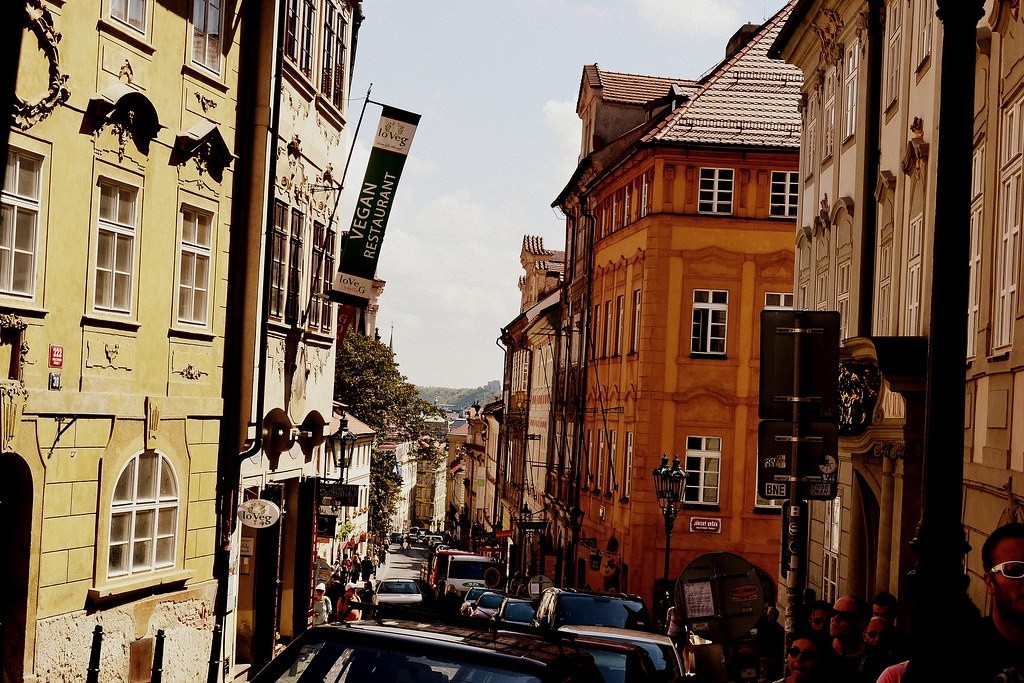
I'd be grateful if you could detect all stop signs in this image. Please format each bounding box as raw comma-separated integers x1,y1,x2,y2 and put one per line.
484,567,500,588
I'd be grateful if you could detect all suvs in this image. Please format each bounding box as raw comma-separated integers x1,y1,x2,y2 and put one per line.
248,599,607,683
389,533,403,545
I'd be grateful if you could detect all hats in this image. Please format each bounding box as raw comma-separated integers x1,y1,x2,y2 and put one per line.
871,591,897,606
315,583,326,591
345,582,358,591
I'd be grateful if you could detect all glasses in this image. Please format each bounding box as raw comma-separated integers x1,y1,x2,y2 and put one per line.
864,630,885,639
811,617,828,624
788,648,816,660
830,608,855,623
983,560,1024,578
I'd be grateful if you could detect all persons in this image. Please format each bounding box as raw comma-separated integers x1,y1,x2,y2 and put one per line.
900,523,1024,683
313,553,374,625
664,607,690,677
771,589,913,683
510,571,530,596
369,537,388,565
757,608,783,683
400,534,411,550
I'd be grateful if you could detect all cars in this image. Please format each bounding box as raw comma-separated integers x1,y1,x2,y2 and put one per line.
372,578,423,618
459,586,690,683
408,528,497,603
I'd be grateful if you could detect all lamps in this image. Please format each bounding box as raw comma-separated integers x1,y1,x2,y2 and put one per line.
317,412,358,484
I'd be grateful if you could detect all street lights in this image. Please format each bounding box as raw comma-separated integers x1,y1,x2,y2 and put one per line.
652,450,690,623
519,501,533,584
